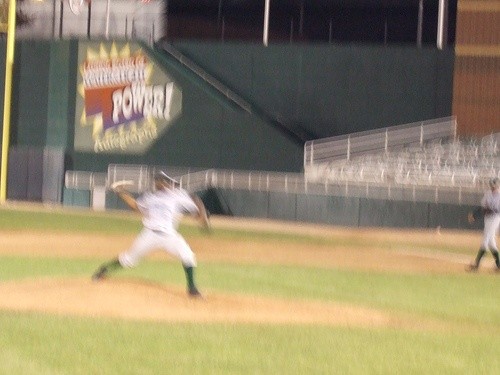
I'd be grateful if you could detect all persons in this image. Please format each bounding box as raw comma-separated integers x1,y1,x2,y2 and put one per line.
91,164,211,296
466,175,499,272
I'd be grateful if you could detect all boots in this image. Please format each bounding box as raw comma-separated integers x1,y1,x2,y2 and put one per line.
493,252,500,271
466,249,486,270
91,256,120,280
184,266,202,297
